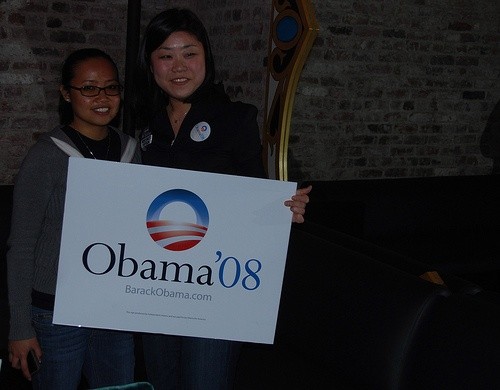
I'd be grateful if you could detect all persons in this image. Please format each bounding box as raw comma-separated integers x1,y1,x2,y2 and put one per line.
136,9,313,390
8,48,140,390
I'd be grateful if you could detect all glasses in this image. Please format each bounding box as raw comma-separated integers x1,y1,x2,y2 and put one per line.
66,83,125,98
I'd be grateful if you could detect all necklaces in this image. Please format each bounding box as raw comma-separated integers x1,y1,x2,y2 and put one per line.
169,107,190,124
76,129,111,160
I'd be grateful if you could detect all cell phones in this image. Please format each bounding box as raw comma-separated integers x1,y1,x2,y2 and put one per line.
26,349,40,377
